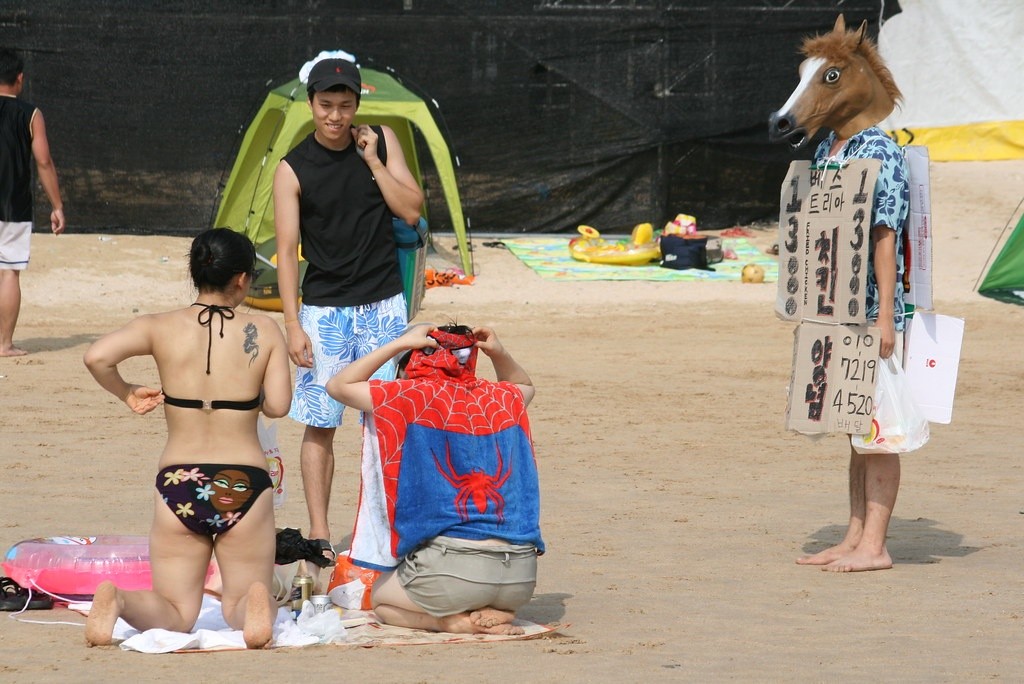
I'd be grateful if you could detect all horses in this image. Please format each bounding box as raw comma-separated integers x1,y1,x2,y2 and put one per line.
768,13,904,151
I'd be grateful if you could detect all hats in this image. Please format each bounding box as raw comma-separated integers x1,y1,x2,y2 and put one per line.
306,57,361,94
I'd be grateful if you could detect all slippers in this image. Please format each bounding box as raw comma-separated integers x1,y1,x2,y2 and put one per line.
306,538,337,567
0,576,56,613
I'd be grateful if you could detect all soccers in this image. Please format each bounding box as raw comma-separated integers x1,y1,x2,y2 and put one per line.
742,264,764,284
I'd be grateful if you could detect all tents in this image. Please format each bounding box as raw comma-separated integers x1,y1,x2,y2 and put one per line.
207,62,475,312
971,198,1024,304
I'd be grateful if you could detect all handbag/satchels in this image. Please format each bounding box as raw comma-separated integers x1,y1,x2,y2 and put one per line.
392,215,430,251
661,232,710,270
851,352,931,455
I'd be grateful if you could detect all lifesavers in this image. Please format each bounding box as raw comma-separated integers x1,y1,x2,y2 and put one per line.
569,223,662,265
3,535,215,595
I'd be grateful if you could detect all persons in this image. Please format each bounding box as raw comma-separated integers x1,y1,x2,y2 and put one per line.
768,13,930,573
273,58,424,565
0,48,67,357
83,228,292,651
324,323,545,635
